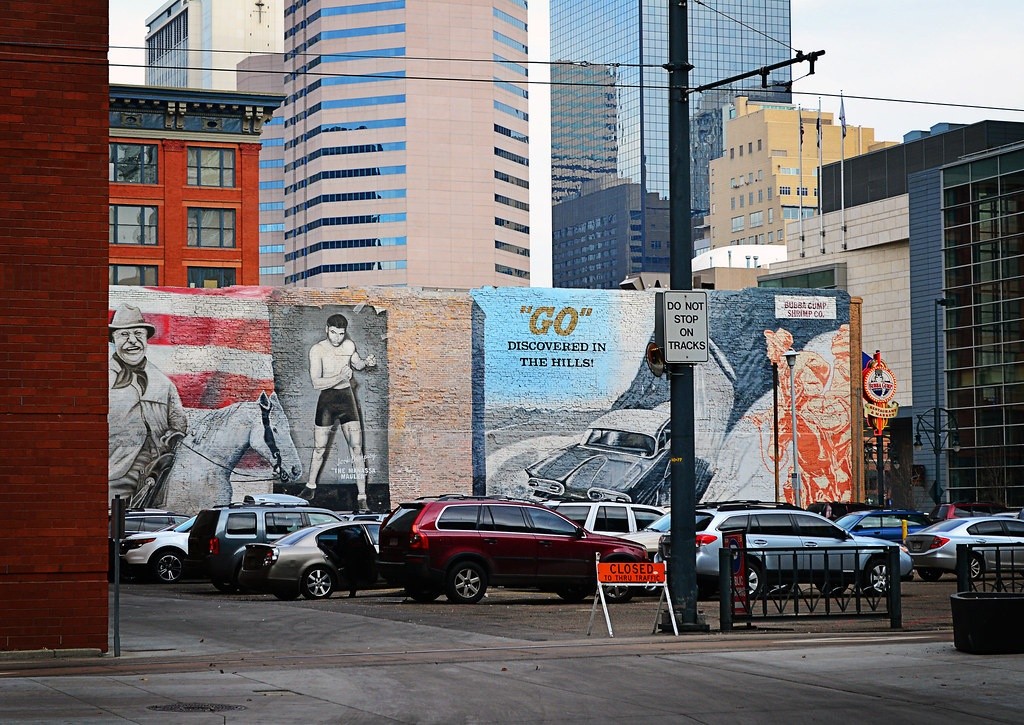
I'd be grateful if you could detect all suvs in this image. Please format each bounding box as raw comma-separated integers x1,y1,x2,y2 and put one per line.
378,493,651,604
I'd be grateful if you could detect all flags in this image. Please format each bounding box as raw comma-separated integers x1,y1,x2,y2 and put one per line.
800,118,804,145
838,90,846,139
816,118,822,148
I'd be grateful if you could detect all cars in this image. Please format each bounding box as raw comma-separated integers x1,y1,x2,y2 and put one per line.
834,509,935,541
906,517,1024,582
237,522,380,599
544,501,913,600
189,505,361,594
108,508,197,583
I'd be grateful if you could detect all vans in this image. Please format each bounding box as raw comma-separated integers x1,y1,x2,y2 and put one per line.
930,502,1024,518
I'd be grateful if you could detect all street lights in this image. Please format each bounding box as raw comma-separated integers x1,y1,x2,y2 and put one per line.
782,347,801,507
913,297,960,508
864,435,901,507
